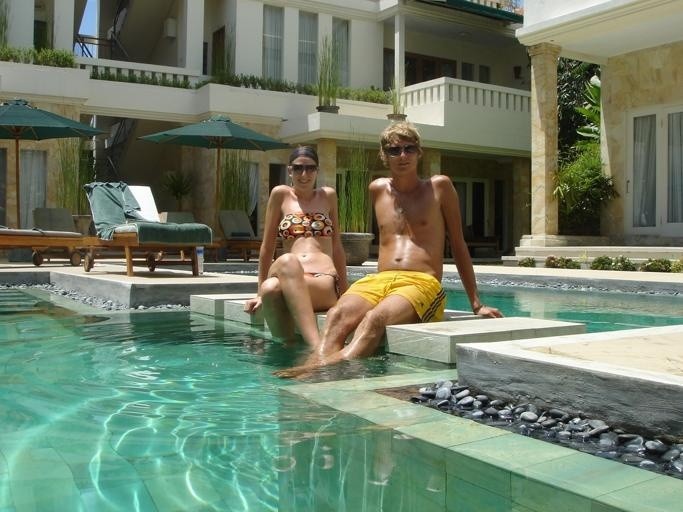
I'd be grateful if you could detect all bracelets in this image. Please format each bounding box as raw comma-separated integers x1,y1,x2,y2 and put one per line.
472,301,486,314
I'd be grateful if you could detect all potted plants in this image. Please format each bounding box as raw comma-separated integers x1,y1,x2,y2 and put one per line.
315,31,345,114
386,76,408,122
334,119,376,266
46,95,98,236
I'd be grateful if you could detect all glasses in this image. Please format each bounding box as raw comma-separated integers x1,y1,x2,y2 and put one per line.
386,145,421,156
291,163,318,175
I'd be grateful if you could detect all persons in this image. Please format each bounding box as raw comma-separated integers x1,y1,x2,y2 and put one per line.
242,145,350,351
271,365,437,492
266,120,504,379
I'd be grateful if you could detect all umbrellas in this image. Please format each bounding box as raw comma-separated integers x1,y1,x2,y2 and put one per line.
0,98,112,229
133,113,295,224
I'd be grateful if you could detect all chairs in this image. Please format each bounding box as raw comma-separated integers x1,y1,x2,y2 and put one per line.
0,182,282,277
